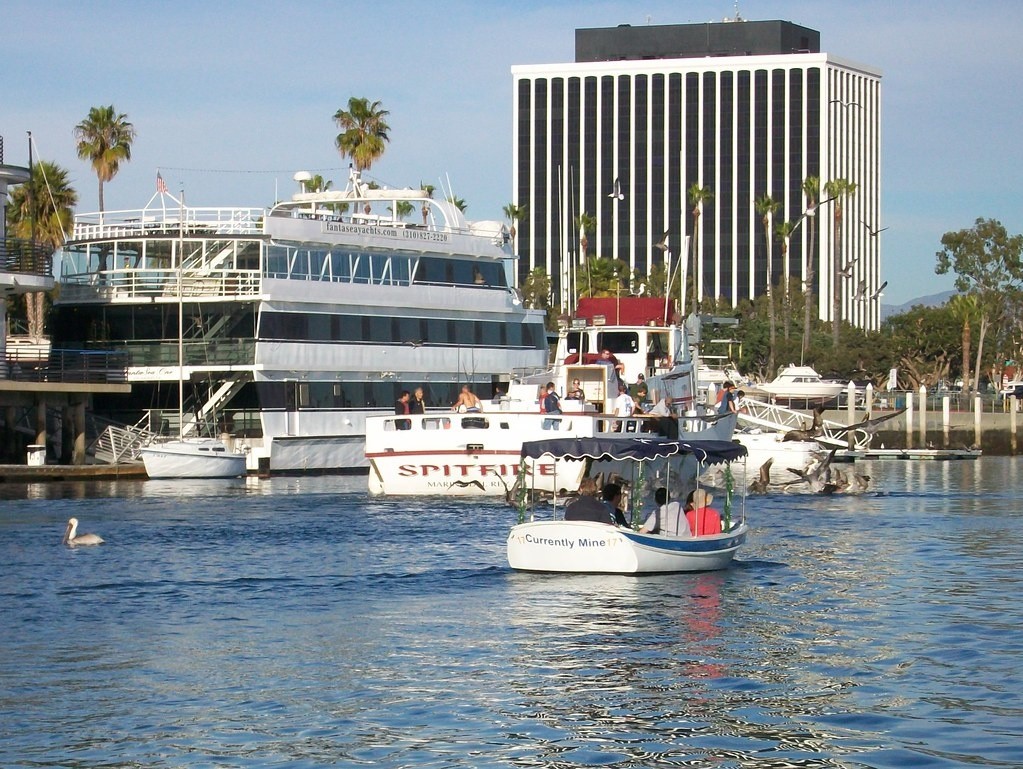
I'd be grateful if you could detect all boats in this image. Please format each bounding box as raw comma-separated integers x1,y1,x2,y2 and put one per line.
757,366,844,403
507,436,749,575
362,249,739,507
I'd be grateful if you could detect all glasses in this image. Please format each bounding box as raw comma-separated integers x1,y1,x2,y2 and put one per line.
639,378,644,380
573,382,579,385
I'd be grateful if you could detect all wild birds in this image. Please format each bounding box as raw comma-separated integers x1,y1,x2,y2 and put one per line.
962,444,982,451
780,405,827,442
829,100,863,109
858,221,887,239
773,447,869,495
472,264,486,285
627,283,647,297
827,407,909,446
491,471,540,510
786,194,838,236
403,340,428,349
880,443,885,449
446,479,485,491
924,441,935,448
62,517,107,548
607,179,624,202
837,258,889,304
746,458,773,498
652,230,671,251
939,424,966,429
188,315,209,327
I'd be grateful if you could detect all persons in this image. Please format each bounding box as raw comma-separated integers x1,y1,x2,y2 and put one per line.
713,380,745,426
595,349,627,395
564,378,585,400
648,396,677,434
611,385,635,432
538,382,563,430
394,390,410,430
492,385,507,404
565,477,613,527
408,387,427,430
450,385,484,429
683,489,722,537
601,484,630,529
636,487,693,537
628,373,649,432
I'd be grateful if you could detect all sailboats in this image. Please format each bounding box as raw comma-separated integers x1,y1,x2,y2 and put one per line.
136,188,249,479
652,236,741,389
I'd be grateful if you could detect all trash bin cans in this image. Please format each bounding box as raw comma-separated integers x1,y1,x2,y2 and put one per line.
26,445,46,466
27,484,49,500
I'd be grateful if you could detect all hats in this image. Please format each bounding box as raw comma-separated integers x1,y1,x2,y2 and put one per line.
638,373,644,379
690,489,713,510
